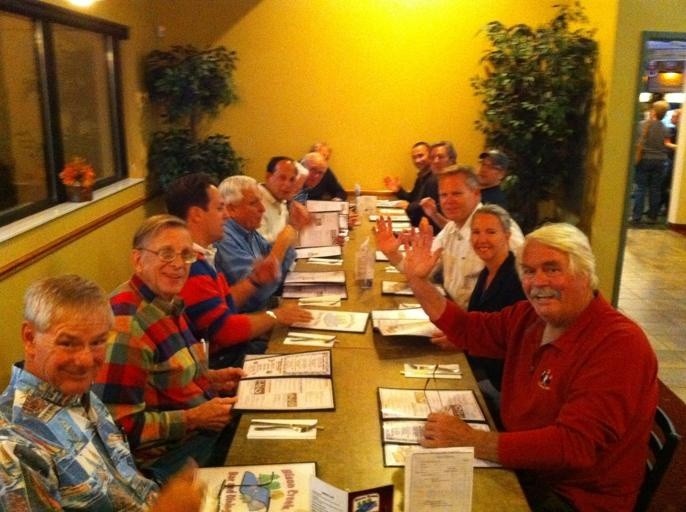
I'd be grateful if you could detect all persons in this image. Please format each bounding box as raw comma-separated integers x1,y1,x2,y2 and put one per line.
87,213,247,489
467,204,528,431
207,174,313,366
251,138,361,273
374,162,526,314
382,141,443,216
0,272,210,511
399,216,661,512
163,169,314,398
625,93,683,227
390,140,458,236
419,148,510,232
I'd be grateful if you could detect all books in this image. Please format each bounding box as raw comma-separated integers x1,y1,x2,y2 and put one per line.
372,250,413,262
367,213,412,232
191,461,318,512
230,349,336,413
375,199,402,208
379,279,448,298
278,269,350,301
374,386,507,470
369,307,443,339
286,308,369,334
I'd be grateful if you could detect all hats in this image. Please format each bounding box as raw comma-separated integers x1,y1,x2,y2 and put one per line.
479,149,510,170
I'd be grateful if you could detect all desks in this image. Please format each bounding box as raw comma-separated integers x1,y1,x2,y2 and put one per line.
214,195,531,512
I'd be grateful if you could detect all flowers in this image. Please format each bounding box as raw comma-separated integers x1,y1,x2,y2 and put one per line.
60,153,97,188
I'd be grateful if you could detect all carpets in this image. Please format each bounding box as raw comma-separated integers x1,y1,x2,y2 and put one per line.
642,377,685,512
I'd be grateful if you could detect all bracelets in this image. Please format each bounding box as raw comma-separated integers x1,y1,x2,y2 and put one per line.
264,310,281,323
246,275,263,292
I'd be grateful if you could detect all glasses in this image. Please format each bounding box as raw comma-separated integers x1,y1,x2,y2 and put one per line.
136,246,198,264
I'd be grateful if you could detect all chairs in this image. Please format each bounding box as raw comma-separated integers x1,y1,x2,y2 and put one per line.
634,406,681,512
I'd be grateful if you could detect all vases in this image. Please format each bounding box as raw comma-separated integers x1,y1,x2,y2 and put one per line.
65,185,92,202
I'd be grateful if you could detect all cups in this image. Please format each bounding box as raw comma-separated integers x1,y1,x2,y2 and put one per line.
358,255,374,289
